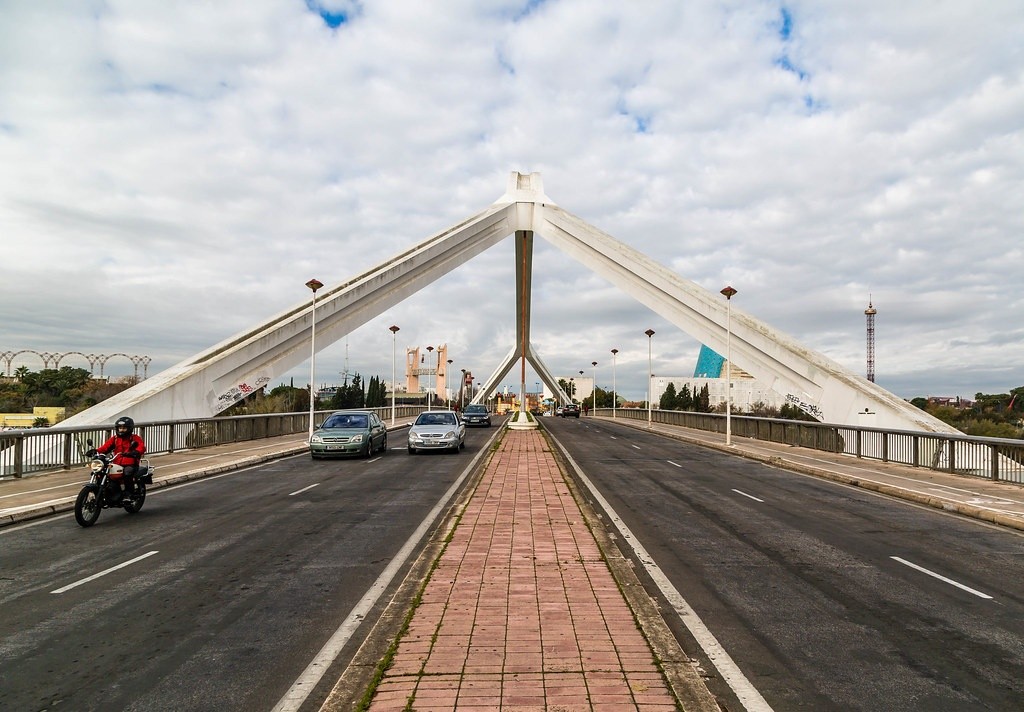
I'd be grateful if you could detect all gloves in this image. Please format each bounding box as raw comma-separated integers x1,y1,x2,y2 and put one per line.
127,450,139,458
86,449,97,457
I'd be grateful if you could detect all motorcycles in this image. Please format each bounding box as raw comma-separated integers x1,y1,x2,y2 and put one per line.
74,439,156,528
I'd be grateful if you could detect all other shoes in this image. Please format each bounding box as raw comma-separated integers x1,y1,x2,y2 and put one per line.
122,499,136,505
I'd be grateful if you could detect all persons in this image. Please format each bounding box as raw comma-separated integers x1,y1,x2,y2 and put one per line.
444,415,451,423
584,404,589,416
479,408,485,413
86,417,145,501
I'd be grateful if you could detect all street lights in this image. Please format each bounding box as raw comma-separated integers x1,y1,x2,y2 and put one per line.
610,349,619,417
579,371,584,415
592,361,597,416
645,328,655,422
304,279,323,443
569,378,574,404
388,324,400,426
426,345,434,411
720,285,738,446
559,388,562,407
447,359,481,410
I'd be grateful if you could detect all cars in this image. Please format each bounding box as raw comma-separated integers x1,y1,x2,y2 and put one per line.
555,407,563,416
310,410,388,461
407,410,466,454
459,404,492,428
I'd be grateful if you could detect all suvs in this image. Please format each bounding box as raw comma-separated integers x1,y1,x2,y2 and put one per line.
562,404,580,418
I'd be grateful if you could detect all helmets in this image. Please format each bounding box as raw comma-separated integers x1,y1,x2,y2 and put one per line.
115,417,134,438
